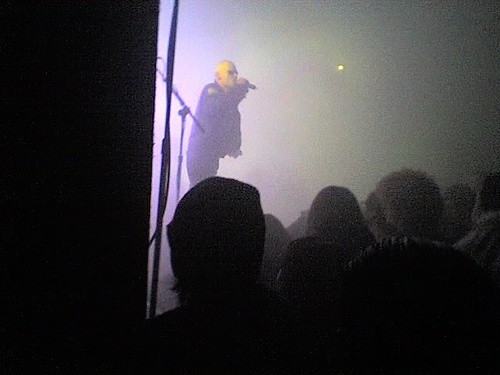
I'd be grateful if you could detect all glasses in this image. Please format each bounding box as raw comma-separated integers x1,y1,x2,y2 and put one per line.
224,70,238,74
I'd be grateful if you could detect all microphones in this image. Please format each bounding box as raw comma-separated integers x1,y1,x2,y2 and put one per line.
236,80,255,90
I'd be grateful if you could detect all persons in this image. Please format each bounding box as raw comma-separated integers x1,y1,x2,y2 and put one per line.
184,59,256,193
133,167,500,374
